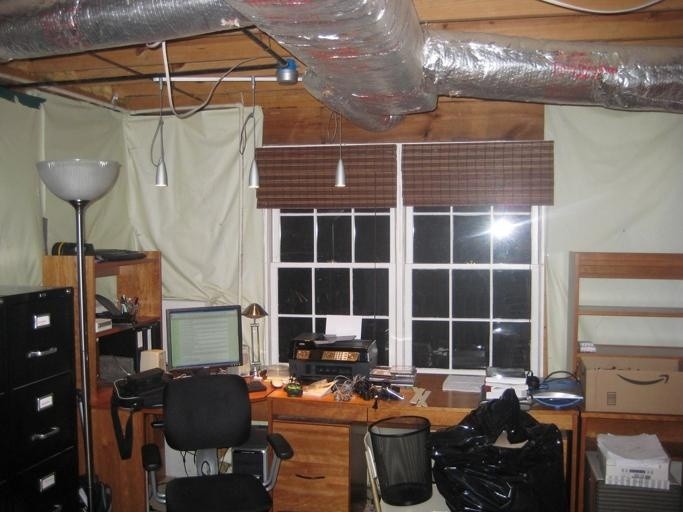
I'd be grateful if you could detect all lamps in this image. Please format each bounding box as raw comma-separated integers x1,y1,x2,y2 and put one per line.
154,81,168,191
34,158,123,512
243,303,269,381
247,76,260,190
332,111,348,188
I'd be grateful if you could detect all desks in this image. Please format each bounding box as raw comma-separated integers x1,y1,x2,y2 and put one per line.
83,381,578,512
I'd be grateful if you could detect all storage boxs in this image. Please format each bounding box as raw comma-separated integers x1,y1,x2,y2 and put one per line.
575,351,683,416
586,434,683,511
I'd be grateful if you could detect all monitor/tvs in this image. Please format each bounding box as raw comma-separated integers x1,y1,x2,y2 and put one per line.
166,305,243,377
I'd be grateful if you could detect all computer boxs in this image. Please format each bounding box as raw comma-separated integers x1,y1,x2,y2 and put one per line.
231,425,268,486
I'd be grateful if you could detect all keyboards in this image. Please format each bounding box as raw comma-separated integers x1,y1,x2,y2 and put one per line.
247,380,267,393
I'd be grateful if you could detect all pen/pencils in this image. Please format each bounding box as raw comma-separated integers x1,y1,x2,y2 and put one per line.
121,294,138,317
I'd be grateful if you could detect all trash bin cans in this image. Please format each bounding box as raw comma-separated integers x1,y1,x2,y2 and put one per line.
368,416,431,507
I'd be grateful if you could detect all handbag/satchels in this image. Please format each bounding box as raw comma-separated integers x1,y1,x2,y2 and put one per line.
111,378,167,408
426,389,563,512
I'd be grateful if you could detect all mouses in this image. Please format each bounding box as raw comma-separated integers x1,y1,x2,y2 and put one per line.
271,376,283,388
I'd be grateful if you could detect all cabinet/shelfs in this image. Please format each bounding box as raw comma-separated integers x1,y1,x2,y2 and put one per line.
0,283,83,510
568,251,683,511
42,248,164,395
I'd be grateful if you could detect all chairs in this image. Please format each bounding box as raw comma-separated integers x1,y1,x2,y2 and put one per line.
141,373,294,510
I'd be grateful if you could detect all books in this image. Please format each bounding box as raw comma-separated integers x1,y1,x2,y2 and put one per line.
443,376,484,392
483,368,529,400
